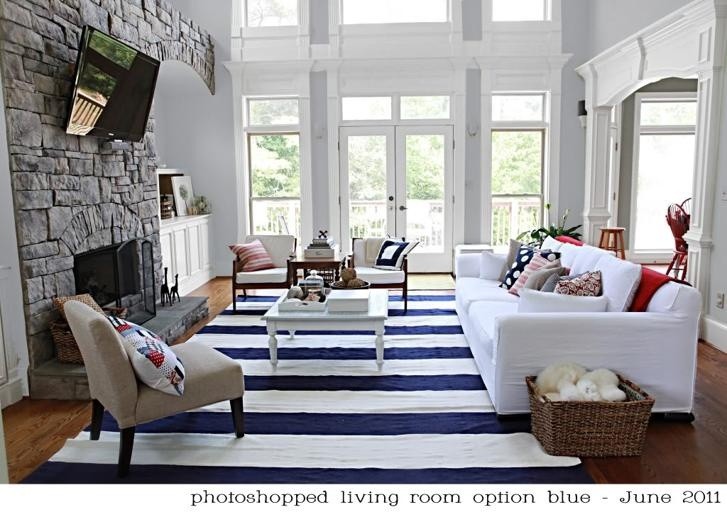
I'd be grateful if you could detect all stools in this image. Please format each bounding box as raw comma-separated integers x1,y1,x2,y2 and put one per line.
597,227,627,257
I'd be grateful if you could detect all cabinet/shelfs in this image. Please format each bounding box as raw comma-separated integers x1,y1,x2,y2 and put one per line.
157,169,217,297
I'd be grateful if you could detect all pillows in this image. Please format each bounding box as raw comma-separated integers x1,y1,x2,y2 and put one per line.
107,317,185,397
496,235,643,311
227,239,276,271
372,238,419,270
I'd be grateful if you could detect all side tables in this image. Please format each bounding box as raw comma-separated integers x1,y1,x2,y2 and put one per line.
291,256,342,286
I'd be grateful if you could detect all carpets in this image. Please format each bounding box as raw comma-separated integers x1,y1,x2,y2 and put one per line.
15,289,588,481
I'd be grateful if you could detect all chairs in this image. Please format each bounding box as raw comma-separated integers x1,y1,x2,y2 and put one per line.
63,300,245,479
233,235,296,312
665,197,697,280
348,237,407,314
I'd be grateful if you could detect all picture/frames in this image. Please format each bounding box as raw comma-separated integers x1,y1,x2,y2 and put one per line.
171,175,195,217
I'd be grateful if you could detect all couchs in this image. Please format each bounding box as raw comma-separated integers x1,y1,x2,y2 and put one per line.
455,235,703,422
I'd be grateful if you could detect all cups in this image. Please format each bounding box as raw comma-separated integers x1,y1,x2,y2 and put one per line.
308,289,322,302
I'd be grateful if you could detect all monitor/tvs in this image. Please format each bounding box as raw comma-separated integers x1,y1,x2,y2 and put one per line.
65,24,161,143
